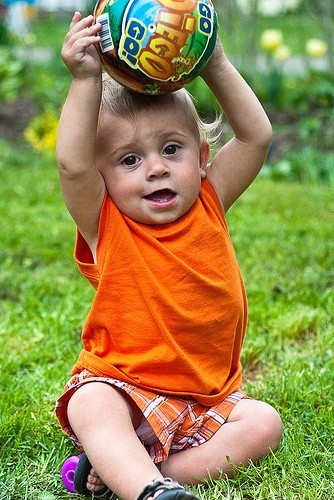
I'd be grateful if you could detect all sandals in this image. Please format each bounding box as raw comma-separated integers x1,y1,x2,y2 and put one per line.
74,453,118,499
138,478,200,500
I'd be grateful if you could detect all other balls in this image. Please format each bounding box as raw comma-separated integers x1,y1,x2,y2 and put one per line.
89,0,218,96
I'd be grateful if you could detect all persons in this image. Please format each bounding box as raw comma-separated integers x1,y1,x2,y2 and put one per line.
56,6,282,500
